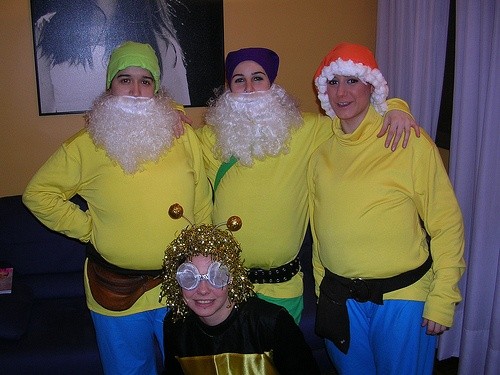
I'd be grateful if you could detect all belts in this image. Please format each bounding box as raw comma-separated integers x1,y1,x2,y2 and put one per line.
245,257,301,284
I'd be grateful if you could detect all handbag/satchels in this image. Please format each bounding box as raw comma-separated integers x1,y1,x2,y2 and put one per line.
83,243,165,311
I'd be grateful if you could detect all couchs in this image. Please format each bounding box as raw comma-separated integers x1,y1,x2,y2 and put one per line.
0,195,338,375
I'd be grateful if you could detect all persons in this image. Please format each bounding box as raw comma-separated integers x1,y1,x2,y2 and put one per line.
158,203,320,375
308,43,466,375
22,42,212,375
168,48,420,326
36,0,191,113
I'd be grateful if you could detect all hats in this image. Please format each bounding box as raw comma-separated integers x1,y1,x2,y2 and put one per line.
314,42,389,117
107,41,160,95
225,48,279,85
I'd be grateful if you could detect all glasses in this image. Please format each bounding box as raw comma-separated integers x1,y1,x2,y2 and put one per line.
175,262,230,290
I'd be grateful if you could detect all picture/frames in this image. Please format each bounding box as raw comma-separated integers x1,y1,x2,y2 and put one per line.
29,0,226,117
